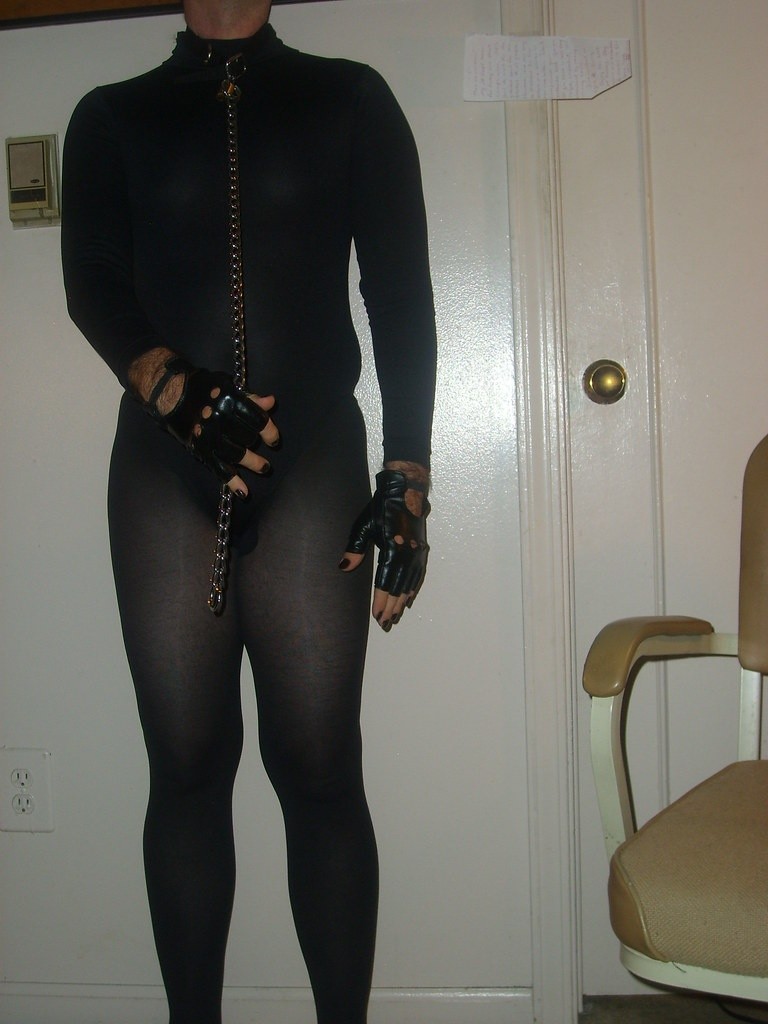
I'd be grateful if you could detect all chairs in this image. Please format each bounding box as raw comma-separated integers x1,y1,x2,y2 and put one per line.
581,436,768,1024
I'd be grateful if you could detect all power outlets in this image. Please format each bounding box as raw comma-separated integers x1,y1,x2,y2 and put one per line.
1,747,53,833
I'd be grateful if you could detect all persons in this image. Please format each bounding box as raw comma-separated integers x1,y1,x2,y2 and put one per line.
60,1,436,1024
